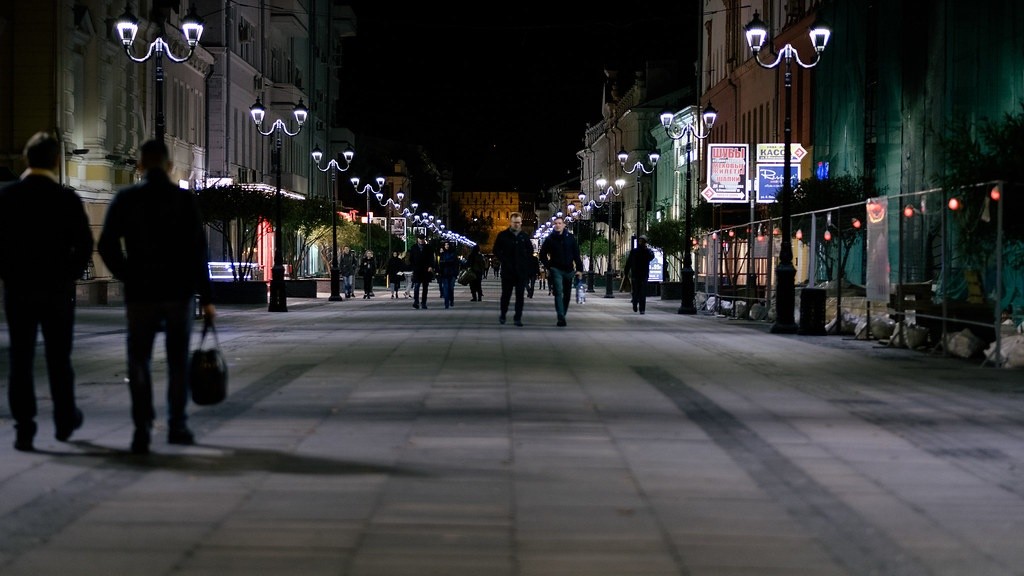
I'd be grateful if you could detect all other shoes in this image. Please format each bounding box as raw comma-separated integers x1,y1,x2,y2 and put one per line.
422,303,427,309
514,319,523,326
15,422,37,450
557,318,566,326
131,430,152,454
413,304,419,309
471,297,477,301
633,303,638,312
450,299,454,306
168,426,194,445
343,288,413,300
56,409,84,441
445,303,450,309
500,316,506,324
640,309,645,314
478,298,481,301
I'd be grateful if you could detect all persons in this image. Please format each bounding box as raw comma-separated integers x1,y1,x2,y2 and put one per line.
492,212,533,326
624,236,655,314
459,246,500,301
360,251,376,299
435,242,459,309
410,234,434,308
0,133,96,449
539,218,583,326
339,246,357,298
96,138,216,452
526,256,555,298
386,252,413,299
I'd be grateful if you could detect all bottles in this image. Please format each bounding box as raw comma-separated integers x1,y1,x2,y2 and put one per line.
576,280,586,305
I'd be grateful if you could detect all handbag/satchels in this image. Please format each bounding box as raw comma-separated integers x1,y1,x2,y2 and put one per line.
457,266,476,286
188,312,228,407
576,279,586,304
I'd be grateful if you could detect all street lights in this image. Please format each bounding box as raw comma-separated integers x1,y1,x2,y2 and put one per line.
742,6,833,335
309,141,355,302
114,0,208,144
349,170,387,296
375,187,406,289
577,186,607,292
594,173,626,299
533,200,592,289
393,199,476,273
659,100,719,316
617,142,662,303
248,93,309,313
